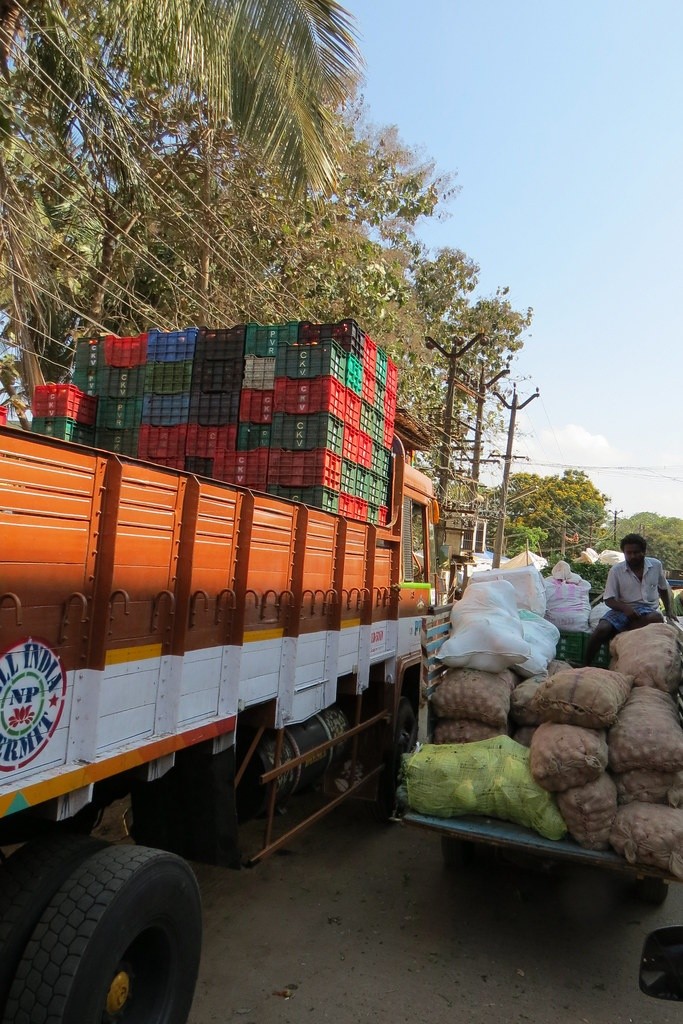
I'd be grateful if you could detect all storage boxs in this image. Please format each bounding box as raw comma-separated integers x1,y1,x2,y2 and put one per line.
146,326,198,361
95,396,143,430
100,366,145,398
141,393,190,426
185,318,397,526
144,359,192,393
141,456,185,470
556,630,582,662
582,632,610,669
74,336,104,368
71,366,100,396
138,422,185,460
32,383,97,426
103,333,148,367
31,417,94,447
94,427,138,459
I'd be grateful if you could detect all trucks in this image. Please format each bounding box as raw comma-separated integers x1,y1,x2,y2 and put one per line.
0,401,472,1024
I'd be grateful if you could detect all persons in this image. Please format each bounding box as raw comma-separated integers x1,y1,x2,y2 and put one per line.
584,532,680,670
